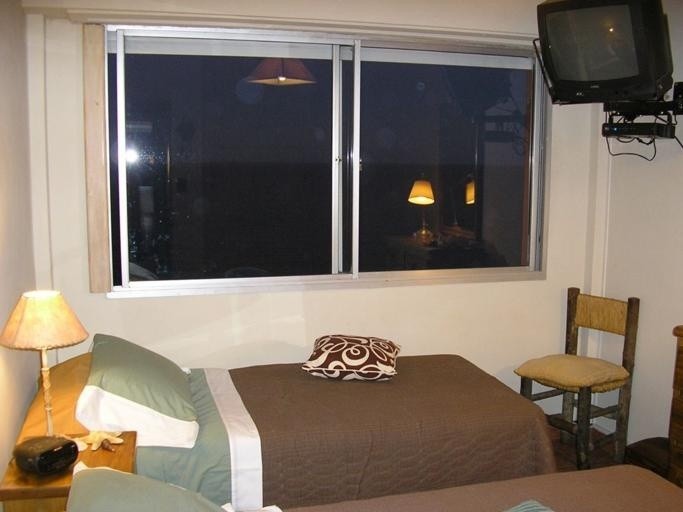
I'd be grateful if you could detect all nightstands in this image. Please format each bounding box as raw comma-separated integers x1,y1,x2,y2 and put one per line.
2,431,136,511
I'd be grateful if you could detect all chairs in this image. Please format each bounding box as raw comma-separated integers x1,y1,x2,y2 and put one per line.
514,288,640,470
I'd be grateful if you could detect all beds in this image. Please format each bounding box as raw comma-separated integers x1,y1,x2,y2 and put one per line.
134,355,556,510
286,462,681,511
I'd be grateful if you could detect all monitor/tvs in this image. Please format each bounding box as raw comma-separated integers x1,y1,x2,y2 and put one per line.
538,0,674,105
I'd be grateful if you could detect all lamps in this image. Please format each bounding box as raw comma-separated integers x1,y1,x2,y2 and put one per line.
0,288,88,442
465,181,477,223
244,60,314,88
406,179,437,245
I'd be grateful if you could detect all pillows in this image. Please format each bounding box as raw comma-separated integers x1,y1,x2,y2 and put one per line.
19,333,198,442
303,334,400,383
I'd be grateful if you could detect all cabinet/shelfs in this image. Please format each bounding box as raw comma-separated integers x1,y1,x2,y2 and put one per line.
667,323,682,487
